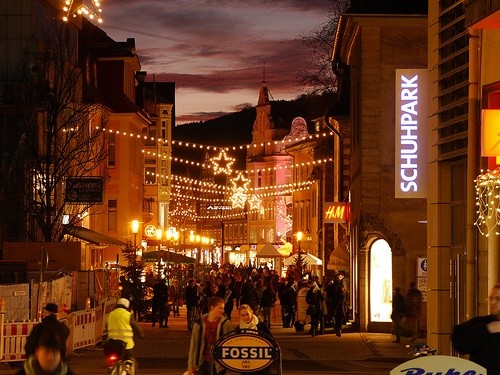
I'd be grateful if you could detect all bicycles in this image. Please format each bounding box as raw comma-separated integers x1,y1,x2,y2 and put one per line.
95,335,145,375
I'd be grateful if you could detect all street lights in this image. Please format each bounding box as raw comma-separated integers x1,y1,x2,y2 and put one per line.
154,227,165,281
127,219,145,321
293,231,304,287
166,229,215,302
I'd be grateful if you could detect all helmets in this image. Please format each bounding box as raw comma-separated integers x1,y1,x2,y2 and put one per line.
117,298,130,308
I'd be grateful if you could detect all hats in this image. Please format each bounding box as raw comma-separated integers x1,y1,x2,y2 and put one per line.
42,303,58,313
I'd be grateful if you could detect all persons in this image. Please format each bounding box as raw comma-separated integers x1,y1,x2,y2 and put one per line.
402,281,423,342
391,287,406,343
451,284,500,375
119,263,350,336
188,296,236,375
24,304,70,360
106,298,144,375
16,334,75,375
235,304,275,375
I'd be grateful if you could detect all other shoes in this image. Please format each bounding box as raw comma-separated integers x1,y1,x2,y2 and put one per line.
391,338,400,343
409,337,417,342
177,313,181,316
158,323,168,328
125,361,132,370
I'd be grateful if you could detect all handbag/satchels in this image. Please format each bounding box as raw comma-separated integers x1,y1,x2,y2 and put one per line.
306,305,316,315
